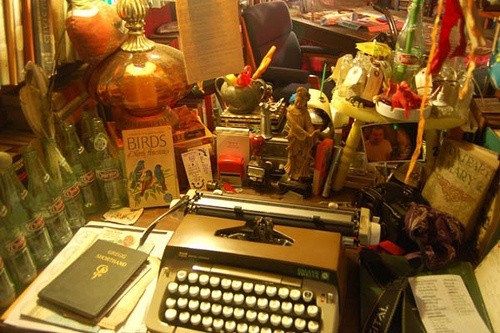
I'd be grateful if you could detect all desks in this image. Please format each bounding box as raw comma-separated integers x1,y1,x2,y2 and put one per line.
286,2,472,66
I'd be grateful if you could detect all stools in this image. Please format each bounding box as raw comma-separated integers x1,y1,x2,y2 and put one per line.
328,88,468,192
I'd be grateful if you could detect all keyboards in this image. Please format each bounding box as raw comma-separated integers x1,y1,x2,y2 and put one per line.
145,256,339,333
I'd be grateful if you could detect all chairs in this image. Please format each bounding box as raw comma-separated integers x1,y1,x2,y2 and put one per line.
243,1,348,105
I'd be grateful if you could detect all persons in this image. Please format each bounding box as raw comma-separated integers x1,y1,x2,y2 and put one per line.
392,127,416,160
283,86,321,183
364,126,392,162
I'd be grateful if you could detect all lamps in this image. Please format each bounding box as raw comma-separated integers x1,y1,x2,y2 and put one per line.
88,1,192,142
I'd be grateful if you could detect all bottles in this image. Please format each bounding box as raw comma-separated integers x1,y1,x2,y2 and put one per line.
391,0,427,95
62,122,104,214
90,117,128,209
0,199,38,285
22,146,73,248
81,111,96,153
41,134,87,229
0,152,54,265
0,254,17,309
